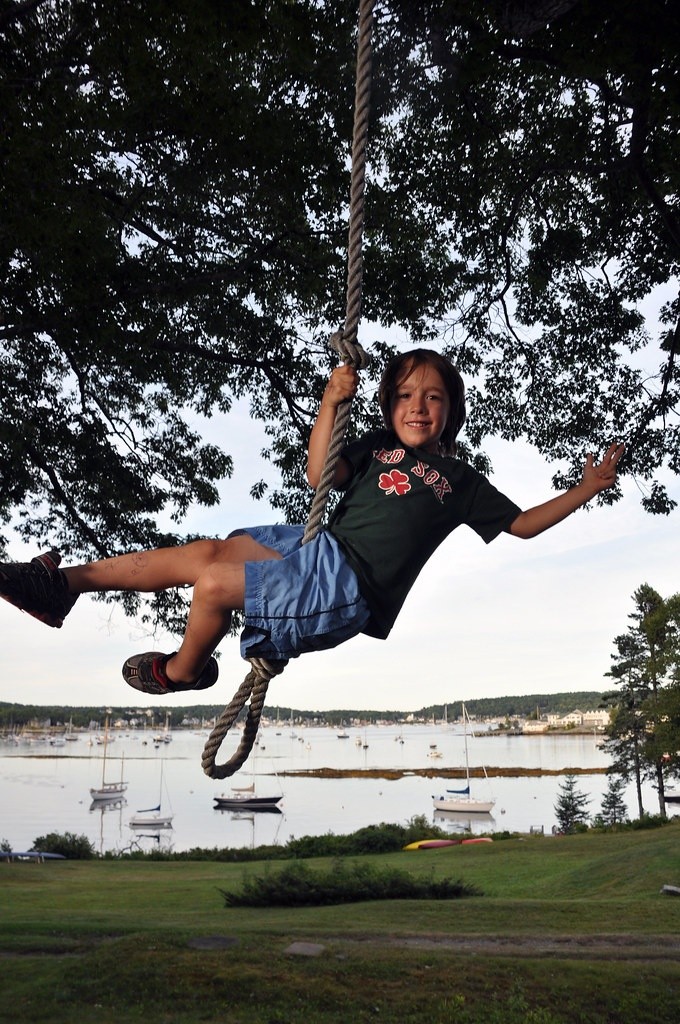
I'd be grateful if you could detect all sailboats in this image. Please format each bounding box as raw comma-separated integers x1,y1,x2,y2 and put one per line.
126,752,176,826
0,704,503,754
128,822,175,854
213,718,285,807
429,701,499,814
89,796,129,855
212,803,285,849
89,713,130,802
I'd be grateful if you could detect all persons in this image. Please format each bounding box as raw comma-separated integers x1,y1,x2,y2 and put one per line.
0,348,625,693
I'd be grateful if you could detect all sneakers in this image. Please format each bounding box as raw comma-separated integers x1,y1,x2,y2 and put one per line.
122,651,219,695
0,551,81,628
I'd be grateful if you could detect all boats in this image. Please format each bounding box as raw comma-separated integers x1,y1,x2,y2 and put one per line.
595,733,680,804
